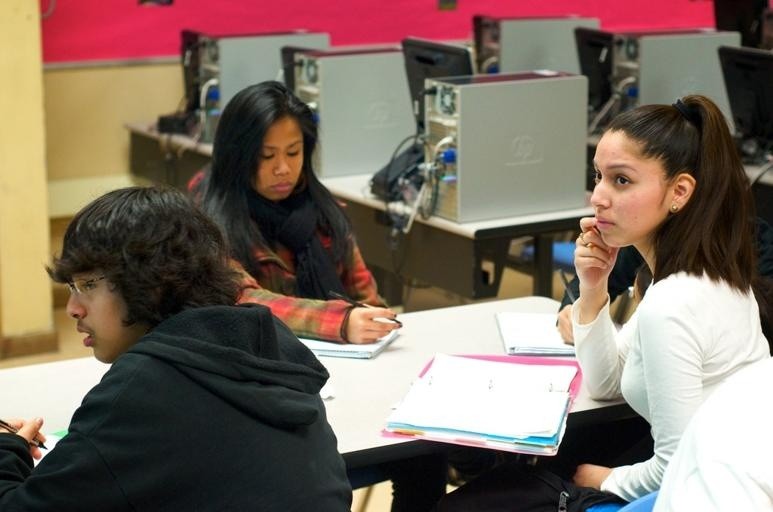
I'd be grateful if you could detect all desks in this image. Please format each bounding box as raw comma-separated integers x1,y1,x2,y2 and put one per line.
0,120,772,511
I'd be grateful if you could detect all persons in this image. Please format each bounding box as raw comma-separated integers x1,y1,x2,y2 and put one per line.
434,92,771,511
556,244,642,345
0,184,352,511
186,81,448,511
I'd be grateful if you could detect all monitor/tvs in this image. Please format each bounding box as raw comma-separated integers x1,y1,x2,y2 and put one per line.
281,46,314,91
371,37,474,201
159,29,202,135
717,45,773,165
574,28,615,125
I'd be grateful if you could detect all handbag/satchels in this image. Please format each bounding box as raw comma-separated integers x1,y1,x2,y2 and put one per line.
371,143,424,201
435,462,616,512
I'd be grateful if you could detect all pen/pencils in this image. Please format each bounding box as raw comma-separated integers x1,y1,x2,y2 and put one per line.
328,290,402,324
0,419,48,449
559,268,576,304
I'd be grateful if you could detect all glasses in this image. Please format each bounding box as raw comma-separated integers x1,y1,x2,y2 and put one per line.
67,276,108,294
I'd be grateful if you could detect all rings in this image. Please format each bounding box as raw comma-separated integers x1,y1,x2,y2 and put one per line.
578,232,587,246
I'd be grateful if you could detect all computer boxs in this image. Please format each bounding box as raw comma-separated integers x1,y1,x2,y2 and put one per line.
197,30,330,143
608,27,742,137
472,15,600,75
292,43,418,180
421,71,591,223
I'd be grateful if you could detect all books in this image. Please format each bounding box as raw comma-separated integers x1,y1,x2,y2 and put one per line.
383,350,581,458
496,311,586,357
296,319,400,359
